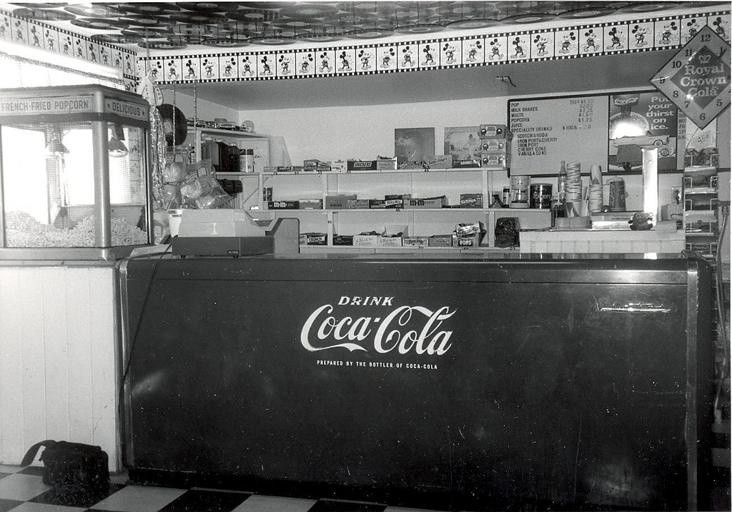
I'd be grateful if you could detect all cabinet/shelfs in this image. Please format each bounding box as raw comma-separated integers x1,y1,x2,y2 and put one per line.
184,122,715,259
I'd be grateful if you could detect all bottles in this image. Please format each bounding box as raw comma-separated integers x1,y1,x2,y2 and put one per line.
224,143,253,173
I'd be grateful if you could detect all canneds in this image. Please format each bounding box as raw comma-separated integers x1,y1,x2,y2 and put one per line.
240,155,255,174
503,175,553,209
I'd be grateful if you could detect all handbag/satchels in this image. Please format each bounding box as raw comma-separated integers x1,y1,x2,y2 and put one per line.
20,439,109,487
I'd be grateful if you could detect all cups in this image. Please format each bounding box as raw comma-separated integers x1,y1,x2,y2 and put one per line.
564,161,626,217
